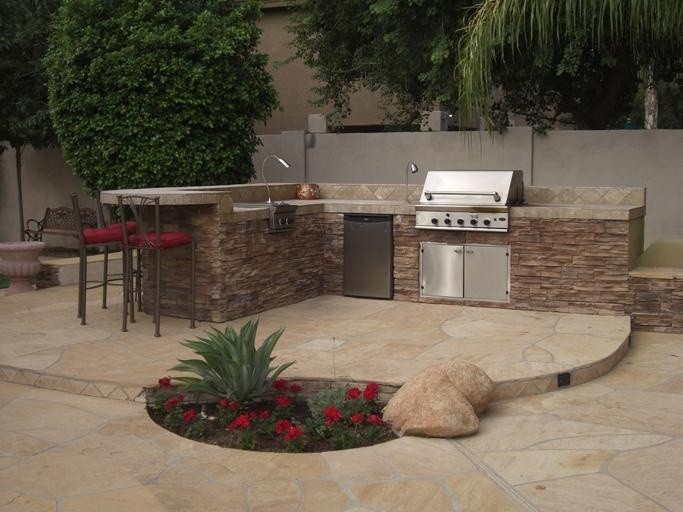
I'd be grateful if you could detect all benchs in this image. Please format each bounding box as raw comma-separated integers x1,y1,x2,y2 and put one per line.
24,206,97,242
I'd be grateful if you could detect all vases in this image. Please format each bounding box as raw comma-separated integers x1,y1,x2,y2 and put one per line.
0,241,46,296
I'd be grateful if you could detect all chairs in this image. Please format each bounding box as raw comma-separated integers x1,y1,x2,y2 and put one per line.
69,189,195,337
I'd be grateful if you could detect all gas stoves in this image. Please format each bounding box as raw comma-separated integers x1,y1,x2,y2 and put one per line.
414,170,526,234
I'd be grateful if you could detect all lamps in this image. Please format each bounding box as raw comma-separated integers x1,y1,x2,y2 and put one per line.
404,160,418,203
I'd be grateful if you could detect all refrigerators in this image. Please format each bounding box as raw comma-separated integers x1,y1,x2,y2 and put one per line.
343,214,393,299
